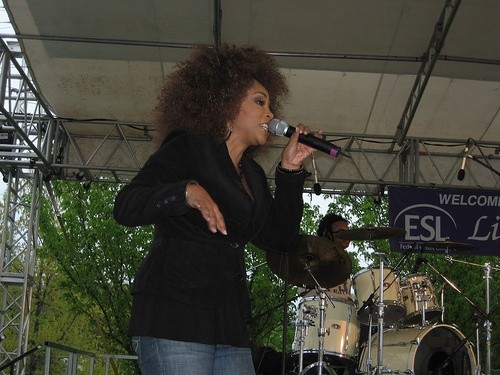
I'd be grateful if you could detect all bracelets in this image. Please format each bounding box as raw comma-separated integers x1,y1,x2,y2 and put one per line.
278,159,305,174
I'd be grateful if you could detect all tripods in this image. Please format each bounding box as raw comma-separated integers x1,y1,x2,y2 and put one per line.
299,264,337,375
370,235,397,375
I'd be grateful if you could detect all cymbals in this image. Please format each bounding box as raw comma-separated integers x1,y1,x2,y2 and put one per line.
266,234,353,289
335,226,407,240
427,240,473,248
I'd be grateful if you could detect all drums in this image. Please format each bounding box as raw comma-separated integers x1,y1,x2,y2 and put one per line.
352,265,405,326
358,323,476,375
291,296,361,369
399,273,442,328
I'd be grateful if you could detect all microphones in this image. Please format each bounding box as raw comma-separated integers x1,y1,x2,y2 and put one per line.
458,140,470,181
268,119,340,157
311,152,321,196
412,245,426,274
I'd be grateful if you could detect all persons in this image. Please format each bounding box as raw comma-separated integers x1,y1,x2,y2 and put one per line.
113,43,324,375
298,215,350,300
